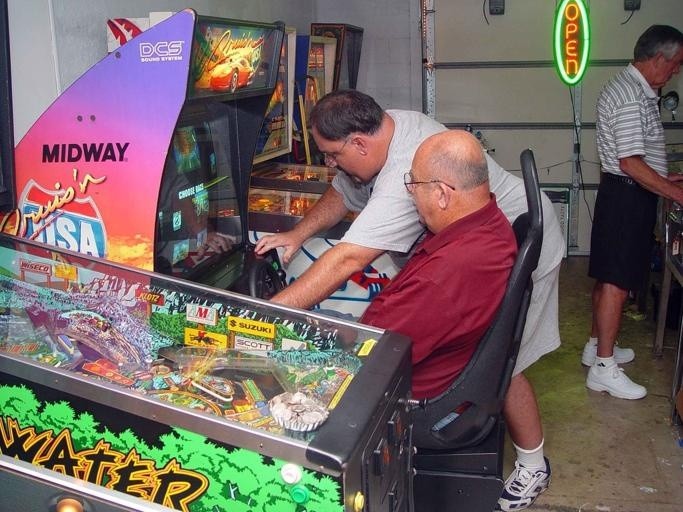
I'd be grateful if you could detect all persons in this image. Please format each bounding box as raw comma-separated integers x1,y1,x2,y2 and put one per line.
582,25,683,400
311,130,519,431
162,159,233,260
254,89,566,512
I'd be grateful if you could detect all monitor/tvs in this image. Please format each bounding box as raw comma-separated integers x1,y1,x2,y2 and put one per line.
156,102,244,281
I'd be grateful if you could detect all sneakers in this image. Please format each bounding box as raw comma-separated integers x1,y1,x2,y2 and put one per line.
586,364,648,402
496,455,551,511
581,339,637,368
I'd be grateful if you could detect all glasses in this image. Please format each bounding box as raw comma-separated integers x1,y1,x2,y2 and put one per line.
403,172,457,195
320,139,350,168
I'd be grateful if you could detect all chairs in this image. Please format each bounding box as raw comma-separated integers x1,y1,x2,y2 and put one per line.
371,146,545,509
